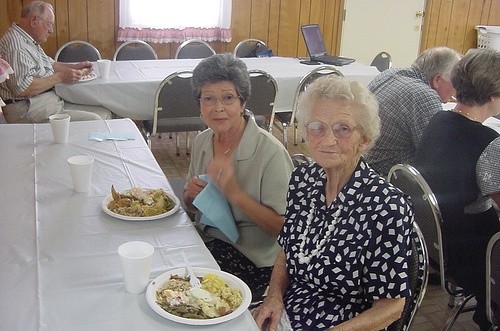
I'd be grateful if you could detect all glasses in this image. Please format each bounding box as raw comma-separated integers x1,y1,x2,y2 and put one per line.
197,93,240,106
305,121,361,138
37,16,54,29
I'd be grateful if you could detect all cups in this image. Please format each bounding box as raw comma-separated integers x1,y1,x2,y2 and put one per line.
68,155,94,192
49,114,71,143
117,241,155,295
96,60,111,79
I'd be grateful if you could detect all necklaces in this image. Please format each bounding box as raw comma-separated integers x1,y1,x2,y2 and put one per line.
453,108,481,123
222,146,233,155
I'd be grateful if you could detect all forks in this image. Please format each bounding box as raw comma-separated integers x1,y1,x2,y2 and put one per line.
181,251,202,288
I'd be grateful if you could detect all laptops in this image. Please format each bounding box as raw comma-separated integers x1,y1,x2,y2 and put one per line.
300,24,354,66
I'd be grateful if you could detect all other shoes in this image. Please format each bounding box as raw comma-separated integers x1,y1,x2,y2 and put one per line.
426,271,442,286
472,301,500,331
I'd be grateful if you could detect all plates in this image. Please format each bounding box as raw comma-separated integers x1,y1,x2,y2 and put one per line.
145,267,253,326
79,74,97,81
101,187,181,221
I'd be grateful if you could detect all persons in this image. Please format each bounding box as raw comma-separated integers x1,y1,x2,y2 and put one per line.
357,47,463,175
183,52,294,301
414,47,500,331
0,1,112,125
251,73,414,331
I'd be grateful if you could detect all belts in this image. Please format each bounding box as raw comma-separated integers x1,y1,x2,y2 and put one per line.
4,86,55,105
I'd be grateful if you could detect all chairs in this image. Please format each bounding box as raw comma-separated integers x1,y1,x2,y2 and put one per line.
53,38,500,331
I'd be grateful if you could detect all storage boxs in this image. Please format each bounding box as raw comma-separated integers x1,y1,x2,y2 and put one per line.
473,24,500,51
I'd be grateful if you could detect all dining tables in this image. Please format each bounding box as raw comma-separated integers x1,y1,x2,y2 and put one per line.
54,55,381,155
0,118,259,331
